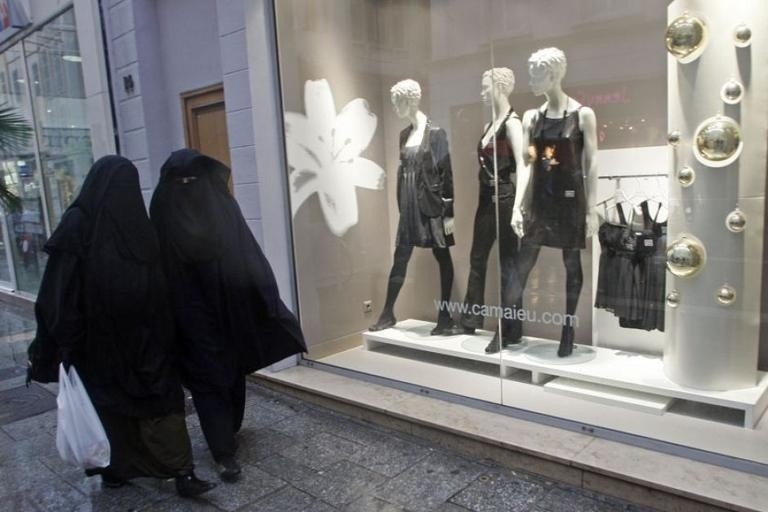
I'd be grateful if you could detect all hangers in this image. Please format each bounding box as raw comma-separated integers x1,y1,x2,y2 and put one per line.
587,172,669,227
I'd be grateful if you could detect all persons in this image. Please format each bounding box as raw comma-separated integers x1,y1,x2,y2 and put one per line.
368,79,456,336
508,45,598,358
443,66,523,352
150,148,306,483
25,154,215,496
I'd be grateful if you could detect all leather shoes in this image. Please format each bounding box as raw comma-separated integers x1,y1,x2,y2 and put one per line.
174,476,217,497
99,471,123,487
218,457,244,477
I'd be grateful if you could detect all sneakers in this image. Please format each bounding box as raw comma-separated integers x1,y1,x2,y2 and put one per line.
368,318,398,332
443,326,475,335
430,318,453,335
486,333,509,353
557,326,575,358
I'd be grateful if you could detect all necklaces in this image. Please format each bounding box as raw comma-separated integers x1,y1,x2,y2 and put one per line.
540,94,568,160
